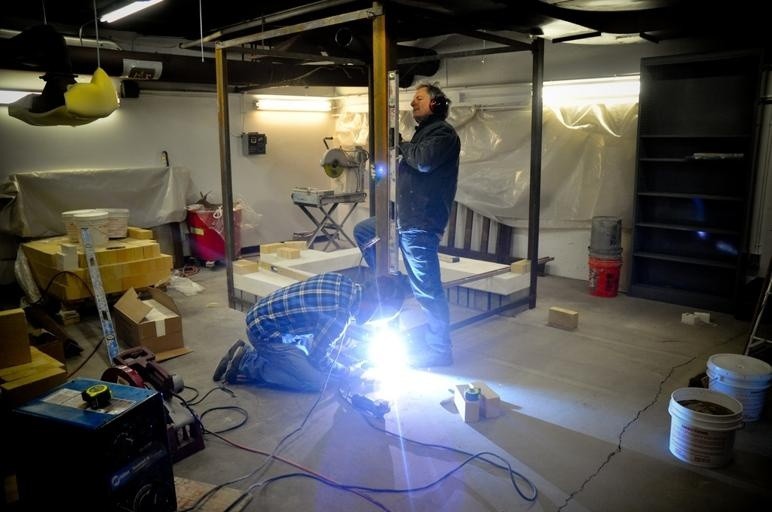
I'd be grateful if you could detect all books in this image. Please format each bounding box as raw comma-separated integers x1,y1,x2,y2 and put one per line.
113,286,196,362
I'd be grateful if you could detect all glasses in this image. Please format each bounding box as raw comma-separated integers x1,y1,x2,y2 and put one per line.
98,0,163,23
253,100,334,111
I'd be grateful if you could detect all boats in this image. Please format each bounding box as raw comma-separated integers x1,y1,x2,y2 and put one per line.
119,79,140,99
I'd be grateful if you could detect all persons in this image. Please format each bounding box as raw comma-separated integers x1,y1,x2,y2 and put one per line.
210,273,404,395
354,79,462,371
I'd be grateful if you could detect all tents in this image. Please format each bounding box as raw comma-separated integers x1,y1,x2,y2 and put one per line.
430,95,450,117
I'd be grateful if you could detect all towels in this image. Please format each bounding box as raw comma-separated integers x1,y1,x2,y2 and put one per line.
187,202,242,262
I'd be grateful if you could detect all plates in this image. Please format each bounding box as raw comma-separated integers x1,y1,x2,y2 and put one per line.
627,46,765,313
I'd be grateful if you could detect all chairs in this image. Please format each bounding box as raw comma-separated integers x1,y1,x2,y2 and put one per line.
212,336,246,382
408,353,453,368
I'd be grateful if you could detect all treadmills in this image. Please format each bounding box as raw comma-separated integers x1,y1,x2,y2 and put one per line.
81,384,112,408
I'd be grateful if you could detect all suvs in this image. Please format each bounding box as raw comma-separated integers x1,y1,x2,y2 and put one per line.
667,387,745,469
589,256,624,297
706,353,772,421
61,209,94,243
97,207,130,238
72,212,109,244
588,215,624,260
